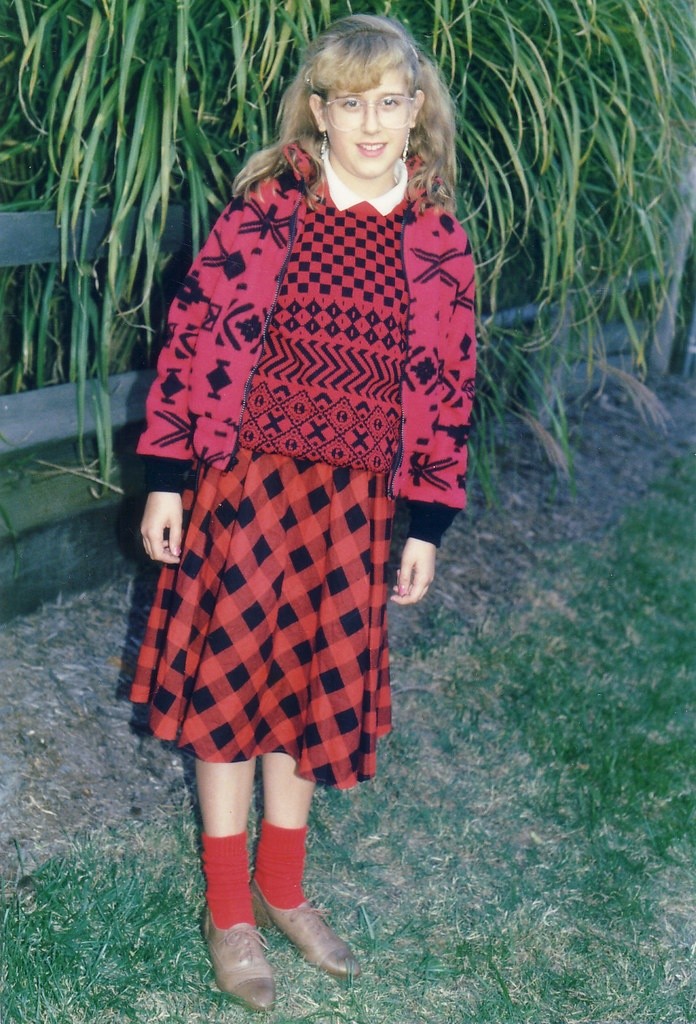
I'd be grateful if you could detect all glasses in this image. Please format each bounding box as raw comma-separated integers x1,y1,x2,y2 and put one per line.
319,95,415,132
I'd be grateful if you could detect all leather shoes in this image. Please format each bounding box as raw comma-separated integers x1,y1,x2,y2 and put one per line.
248,879,361,980
201,908,275,1010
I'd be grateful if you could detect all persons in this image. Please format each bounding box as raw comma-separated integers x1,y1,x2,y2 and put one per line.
126,14,477,1013
